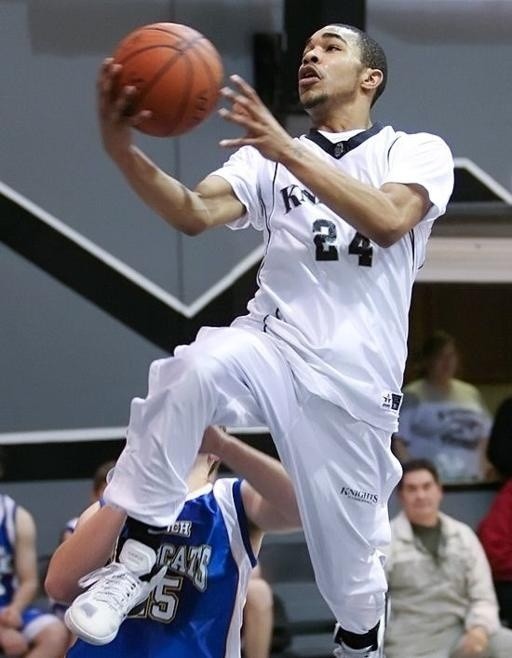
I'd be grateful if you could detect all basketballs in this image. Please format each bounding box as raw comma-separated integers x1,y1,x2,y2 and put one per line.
110,21,224,138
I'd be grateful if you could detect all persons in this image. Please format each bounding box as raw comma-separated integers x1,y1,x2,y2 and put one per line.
379,460,511,658
41,424,304,657
62,22,456,657
391,328,495,484
238,557,274,657
471,391,512,630
64,456,120,537
1,492,73,658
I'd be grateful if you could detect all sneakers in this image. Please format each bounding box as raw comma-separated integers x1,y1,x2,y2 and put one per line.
63,537,171,646
333,596,390,658
